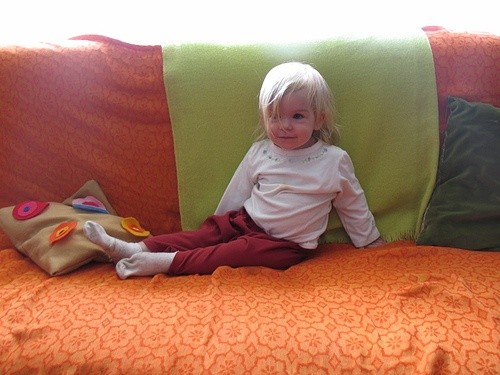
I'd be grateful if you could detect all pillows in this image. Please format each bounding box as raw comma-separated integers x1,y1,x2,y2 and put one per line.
0,178,156,276
416,96,500,252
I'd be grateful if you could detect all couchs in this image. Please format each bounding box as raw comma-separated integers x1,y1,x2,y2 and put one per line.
0,24,500,375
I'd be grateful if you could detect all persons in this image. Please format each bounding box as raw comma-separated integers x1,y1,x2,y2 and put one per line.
86,61,385,279
